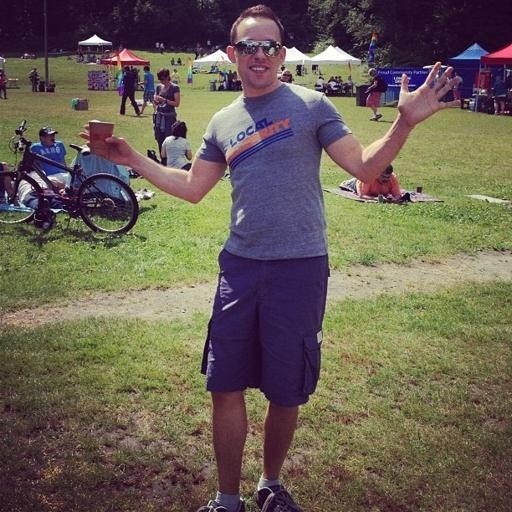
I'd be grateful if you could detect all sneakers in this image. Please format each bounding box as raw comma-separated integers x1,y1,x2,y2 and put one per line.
196,497,248,512
373,113,382,119
254,483,302,512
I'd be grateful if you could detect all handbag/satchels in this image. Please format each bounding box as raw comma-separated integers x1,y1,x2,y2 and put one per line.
374,77,387,92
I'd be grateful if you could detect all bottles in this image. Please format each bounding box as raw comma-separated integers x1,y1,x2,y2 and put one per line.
73,174,80,198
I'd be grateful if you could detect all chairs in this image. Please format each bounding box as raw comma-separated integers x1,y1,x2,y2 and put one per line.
315,82,355,97
211,79,244,92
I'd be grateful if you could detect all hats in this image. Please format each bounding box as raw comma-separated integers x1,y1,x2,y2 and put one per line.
38,126,58,137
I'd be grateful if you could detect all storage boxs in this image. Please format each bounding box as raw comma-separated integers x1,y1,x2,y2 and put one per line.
73,99,88,110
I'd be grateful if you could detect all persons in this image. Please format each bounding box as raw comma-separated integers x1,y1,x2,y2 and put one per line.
339,163,402,202
278,64,512,124
28,68,41,92
118,37,243,173
75,45,113,68
0,68,9,99
0,125,71,218
79,5,463,512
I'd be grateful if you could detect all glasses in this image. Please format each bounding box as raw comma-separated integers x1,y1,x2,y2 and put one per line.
233,38,284,56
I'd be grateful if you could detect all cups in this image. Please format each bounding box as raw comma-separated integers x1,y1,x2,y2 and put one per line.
89,121,115,156
416,187,421,193
378,195,383,204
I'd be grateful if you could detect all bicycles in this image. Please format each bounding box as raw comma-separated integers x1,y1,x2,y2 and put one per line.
0,121,142,238
